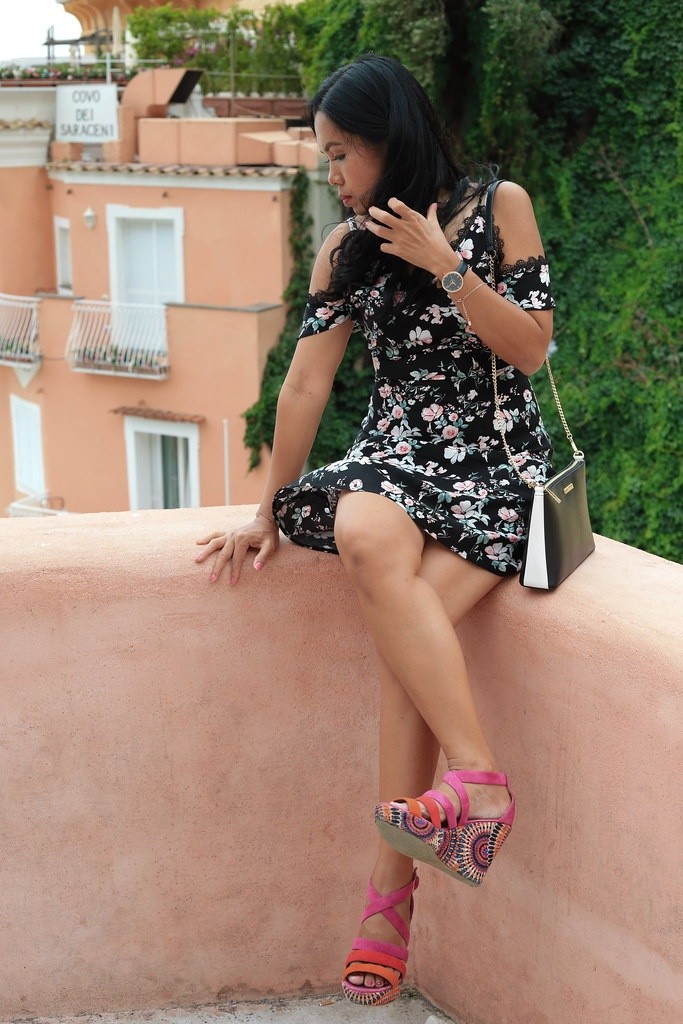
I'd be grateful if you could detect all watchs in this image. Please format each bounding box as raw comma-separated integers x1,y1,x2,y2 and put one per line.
435,260,468,295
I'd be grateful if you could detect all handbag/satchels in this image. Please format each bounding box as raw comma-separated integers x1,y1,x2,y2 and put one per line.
517,449,596,592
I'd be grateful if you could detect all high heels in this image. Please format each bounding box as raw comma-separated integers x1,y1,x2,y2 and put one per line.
374,770,513,885
343,870,416,1003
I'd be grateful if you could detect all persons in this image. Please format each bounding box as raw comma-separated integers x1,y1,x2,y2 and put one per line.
191,59,558,1008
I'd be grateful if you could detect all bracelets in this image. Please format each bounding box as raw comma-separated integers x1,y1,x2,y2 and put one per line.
451,282,488,329
